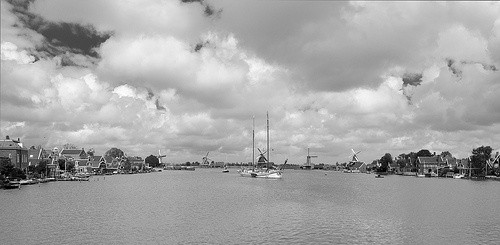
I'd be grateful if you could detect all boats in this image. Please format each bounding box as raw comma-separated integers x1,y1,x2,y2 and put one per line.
222,170,230,173
375,175,384,178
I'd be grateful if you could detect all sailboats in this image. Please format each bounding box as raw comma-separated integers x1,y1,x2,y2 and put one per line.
238,112,283,180
